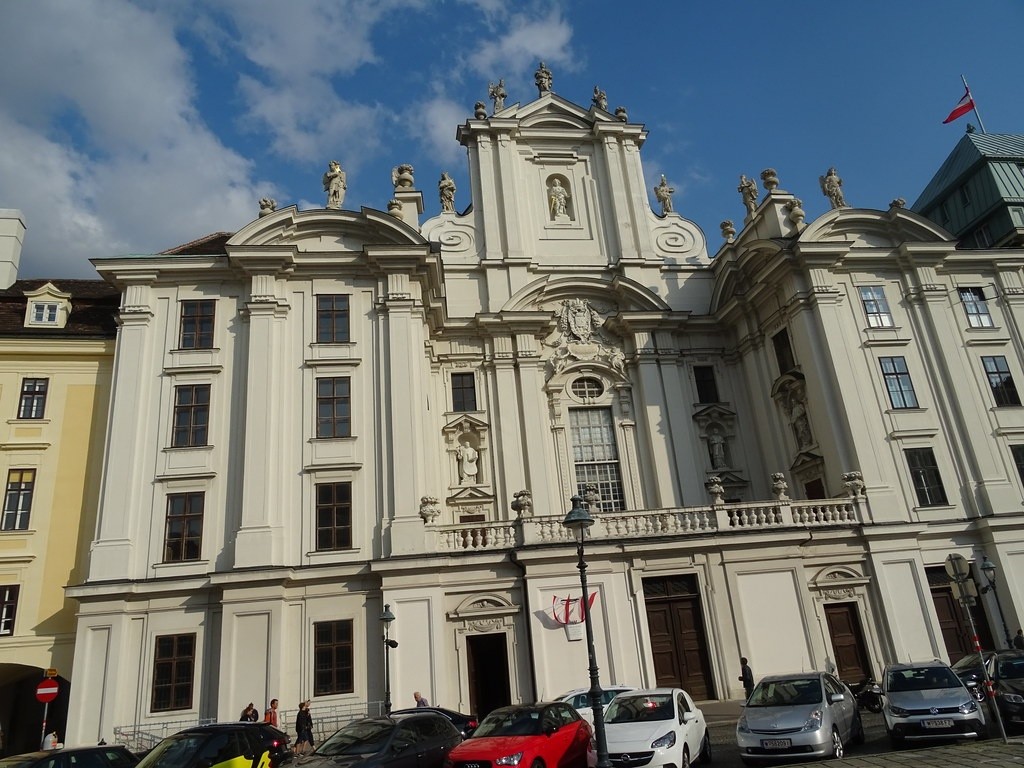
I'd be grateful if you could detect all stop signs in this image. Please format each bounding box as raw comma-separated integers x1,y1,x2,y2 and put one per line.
35,680,60,702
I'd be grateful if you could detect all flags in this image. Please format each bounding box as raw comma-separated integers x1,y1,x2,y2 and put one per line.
943,87,976,124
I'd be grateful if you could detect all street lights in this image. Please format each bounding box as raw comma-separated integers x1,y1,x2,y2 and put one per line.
981,555,1016,649
565,494,615,768
379,603,395,713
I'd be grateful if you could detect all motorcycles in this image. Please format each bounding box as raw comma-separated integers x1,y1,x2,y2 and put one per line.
830,667,882,713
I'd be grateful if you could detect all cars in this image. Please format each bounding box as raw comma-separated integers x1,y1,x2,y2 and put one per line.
588,689,712,768
396,707,479,739
278,713,460,768
448,702,593,767
506,689,657,749
735,673,865,764
0,722,294,768
984,648,1024,731
951,651,997,703
881,660,989,750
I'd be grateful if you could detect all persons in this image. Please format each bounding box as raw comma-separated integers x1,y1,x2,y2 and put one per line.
658,180,673,212
460,442,478,481
554,350,569,374
293,700,316,757
324,161,347,208
1014,629,1024,650
608,347,625,374
534,62,552,90
824,168,846,206
98,738,107,745
440,173,456,212
265,699,279,727
492,80,507,112
239,704,259,722
710,428,726,469
594,87,606,109
739,175,759,214
739,658,754,706
550,179,570,216
414,692,429,707
788,399,812,448
